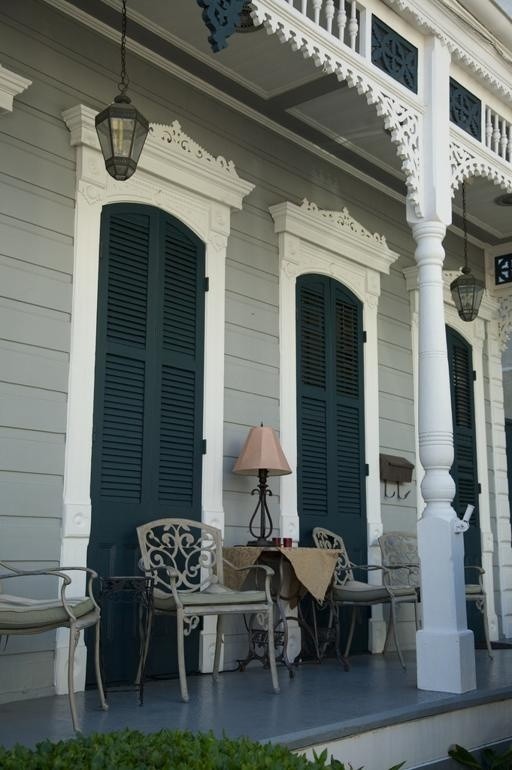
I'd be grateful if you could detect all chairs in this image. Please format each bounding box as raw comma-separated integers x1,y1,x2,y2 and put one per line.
133,518,281,703
312,526,420,671
0,561,109,734
378,534,495,661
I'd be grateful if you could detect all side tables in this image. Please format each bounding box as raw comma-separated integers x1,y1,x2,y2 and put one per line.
93,573,154,707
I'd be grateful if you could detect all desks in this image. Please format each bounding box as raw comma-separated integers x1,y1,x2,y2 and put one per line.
211,546,343,680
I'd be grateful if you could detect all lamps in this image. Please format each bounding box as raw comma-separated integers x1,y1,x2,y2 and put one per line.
450,180,485,322
94,0,150,182
232,421,293,548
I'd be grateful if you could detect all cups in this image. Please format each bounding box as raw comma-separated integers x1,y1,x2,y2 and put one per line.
272,537,293,547
